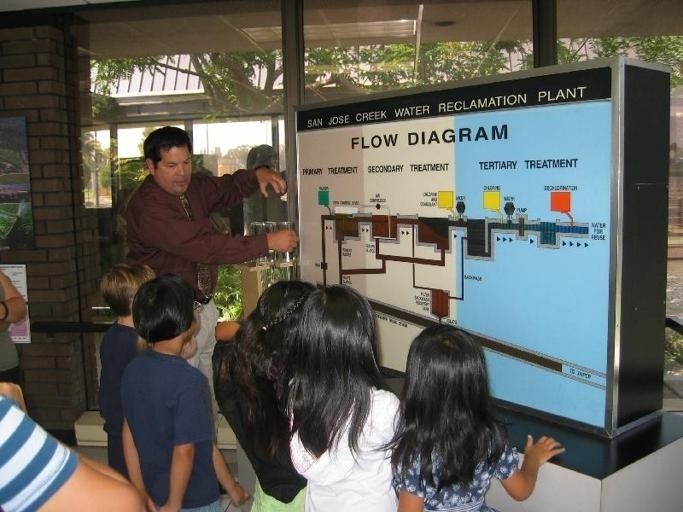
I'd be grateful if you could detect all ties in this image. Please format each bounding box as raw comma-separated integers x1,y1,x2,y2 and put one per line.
180,195,213,299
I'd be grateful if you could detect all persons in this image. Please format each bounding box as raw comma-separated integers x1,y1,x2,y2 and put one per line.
97,259,401,510
1,259,143,512
123,126,300,444
373,328,569,510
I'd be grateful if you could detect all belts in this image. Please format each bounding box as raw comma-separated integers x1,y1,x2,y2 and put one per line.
194,295,212,304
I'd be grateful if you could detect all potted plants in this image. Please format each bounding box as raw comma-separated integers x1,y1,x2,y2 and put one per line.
215,264,242,341
